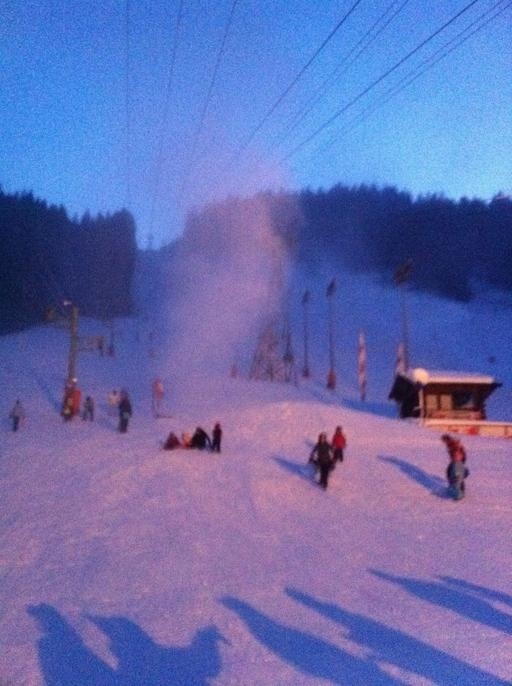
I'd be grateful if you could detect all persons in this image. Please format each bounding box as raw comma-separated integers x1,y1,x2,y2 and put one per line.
162,421,223,454
330,426,347,464
106,389,121,416
81,394,96,421
117,392,134,433
447,449,470,499
307,433,335,490
9,399,23,433
153,376,167,418
441,434,466,494
